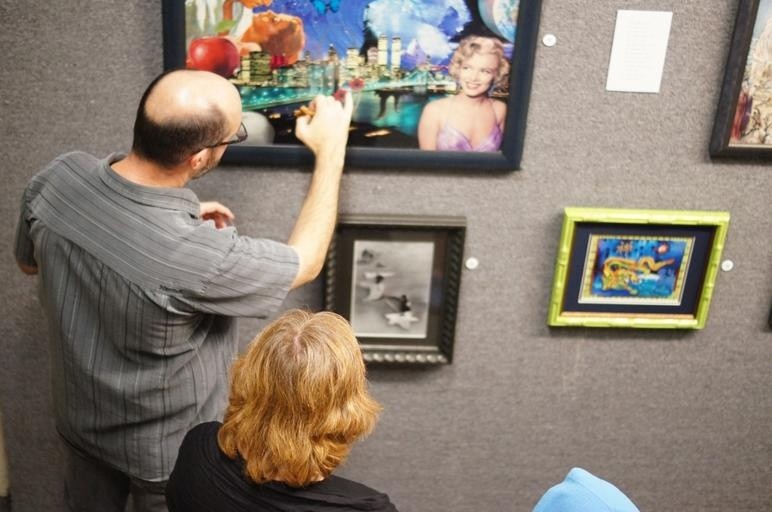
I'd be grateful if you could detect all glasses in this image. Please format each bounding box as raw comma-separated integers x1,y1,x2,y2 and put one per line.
191,122,248,155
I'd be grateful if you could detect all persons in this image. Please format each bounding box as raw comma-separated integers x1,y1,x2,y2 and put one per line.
416,35,510,152
16,68,351,508
166,311,401,511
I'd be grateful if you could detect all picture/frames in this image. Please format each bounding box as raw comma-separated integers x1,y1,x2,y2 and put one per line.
156,0,542,177
545,204,730,335
323,211,466,369
705,0,772,163
